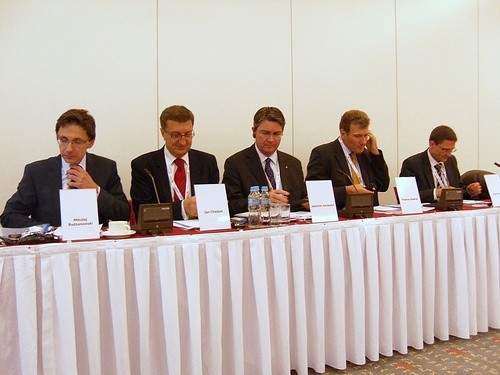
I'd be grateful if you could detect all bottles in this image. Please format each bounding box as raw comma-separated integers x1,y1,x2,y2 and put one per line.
248,186,270,228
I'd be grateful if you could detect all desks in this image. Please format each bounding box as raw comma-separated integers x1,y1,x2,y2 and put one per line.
0,200,499,375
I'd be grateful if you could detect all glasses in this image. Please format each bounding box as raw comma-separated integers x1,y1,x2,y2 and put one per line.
164,129,195,139
57,134,90,147
255,128,283,140
437,145,456,153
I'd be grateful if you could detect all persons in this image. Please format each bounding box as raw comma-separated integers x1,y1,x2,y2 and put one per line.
0,109,130,231
305,110,391,208
222,107,310,217
399,126,482,203
130,106,220,225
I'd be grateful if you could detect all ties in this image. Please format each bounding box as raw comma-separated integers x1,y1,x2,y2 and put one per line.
434,163,444,189
174,159,187,203
264,157,276,190
69,165,82,189
349,153,360,185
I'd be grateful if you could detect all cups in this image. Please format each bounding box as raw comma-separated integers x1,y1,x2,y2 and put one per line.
269,203,290,227
108,221,130,234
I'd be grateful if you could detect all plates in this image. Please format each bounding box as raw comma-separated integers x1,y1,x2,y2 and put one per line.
101,230,136,238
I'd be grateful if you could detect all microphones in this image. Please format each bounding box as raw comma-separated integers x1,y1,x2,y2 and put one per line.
336,168,359,193
436,166,449,186
495,163,500,167
144,168,160,204
